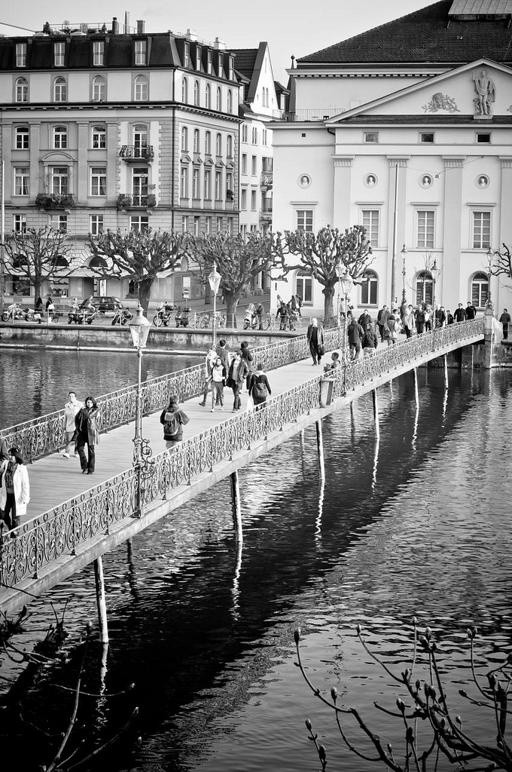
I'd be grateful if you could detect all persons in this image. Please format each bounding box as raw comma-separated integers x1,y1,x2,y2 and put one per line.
471,69,494,116
0,436,9,531
346,299,477,361
61,391,85,459
74,396,100,476
160,395,191,452
498,308,511,340
0,447,31,539
197,339,273,415
307,317,326,367
331,352,341,369
324,361,333,372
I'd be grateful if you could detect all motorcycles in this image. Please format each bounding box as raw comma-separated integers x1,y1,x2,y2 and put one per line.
153,300,173,327
243,303,258,330
111,306,132,326
175,305,192,328
66,295,98,325
2,303,64,323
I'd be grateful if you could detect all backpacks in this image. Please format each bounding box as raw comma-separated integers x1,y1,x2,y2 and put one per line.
163,408,180,436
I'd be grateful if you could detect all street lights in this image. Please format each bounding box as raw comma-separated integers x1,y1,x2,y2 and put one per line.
429,259,440,352
128,302,154,518
334,259,353,397
207,261,222,351
486,247,494,316
401,243,407,304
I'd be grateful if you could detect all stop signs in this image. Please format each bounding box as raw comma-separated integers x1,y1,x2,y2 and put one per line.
182,288,190,295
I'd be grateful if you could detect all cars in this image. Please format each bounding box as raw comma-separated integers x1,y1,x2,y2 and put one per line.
99,297,122,311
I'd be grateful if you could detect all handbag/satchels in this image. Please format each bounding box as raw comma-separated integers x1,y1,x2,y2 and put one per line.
318,344,325,355
254,383,269,397
